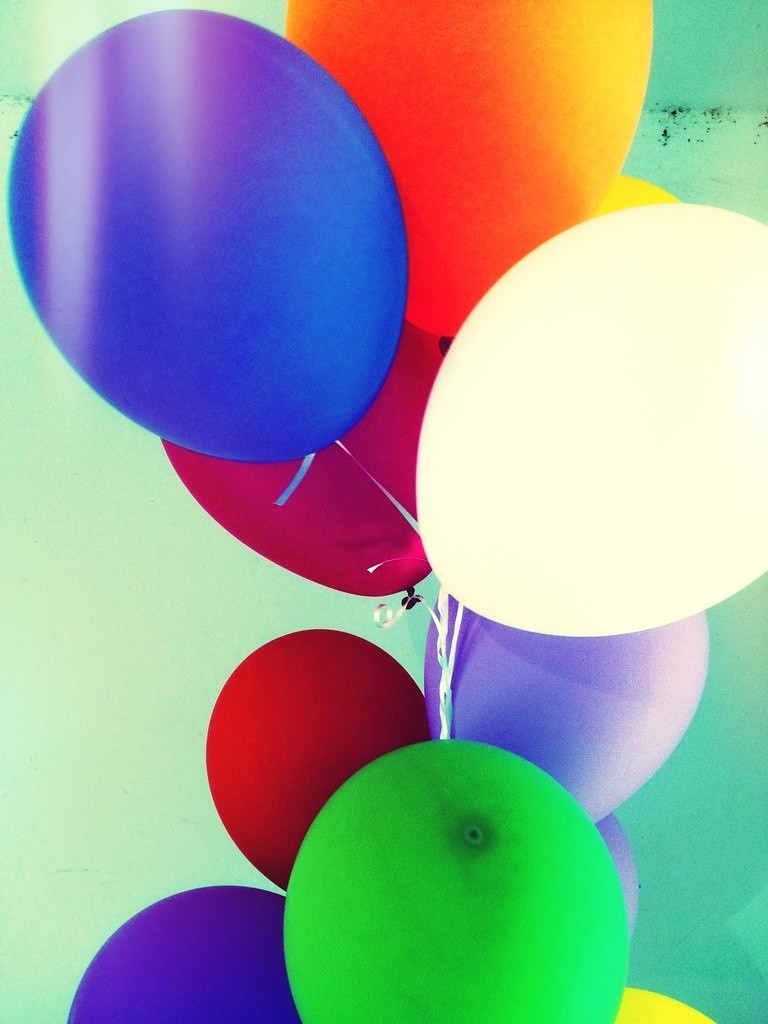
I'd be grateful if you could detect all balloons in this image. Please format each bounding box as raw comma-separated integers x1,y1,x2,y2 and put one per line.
617,987,716,1024
9,9,410,463
284,0,656,352
416,204,768,637
206,630,433,891
161,318,446,608
67,885,303,1023
282,742,628,1024
592,177,680,218
595,814,640,938
425,581,709,824
